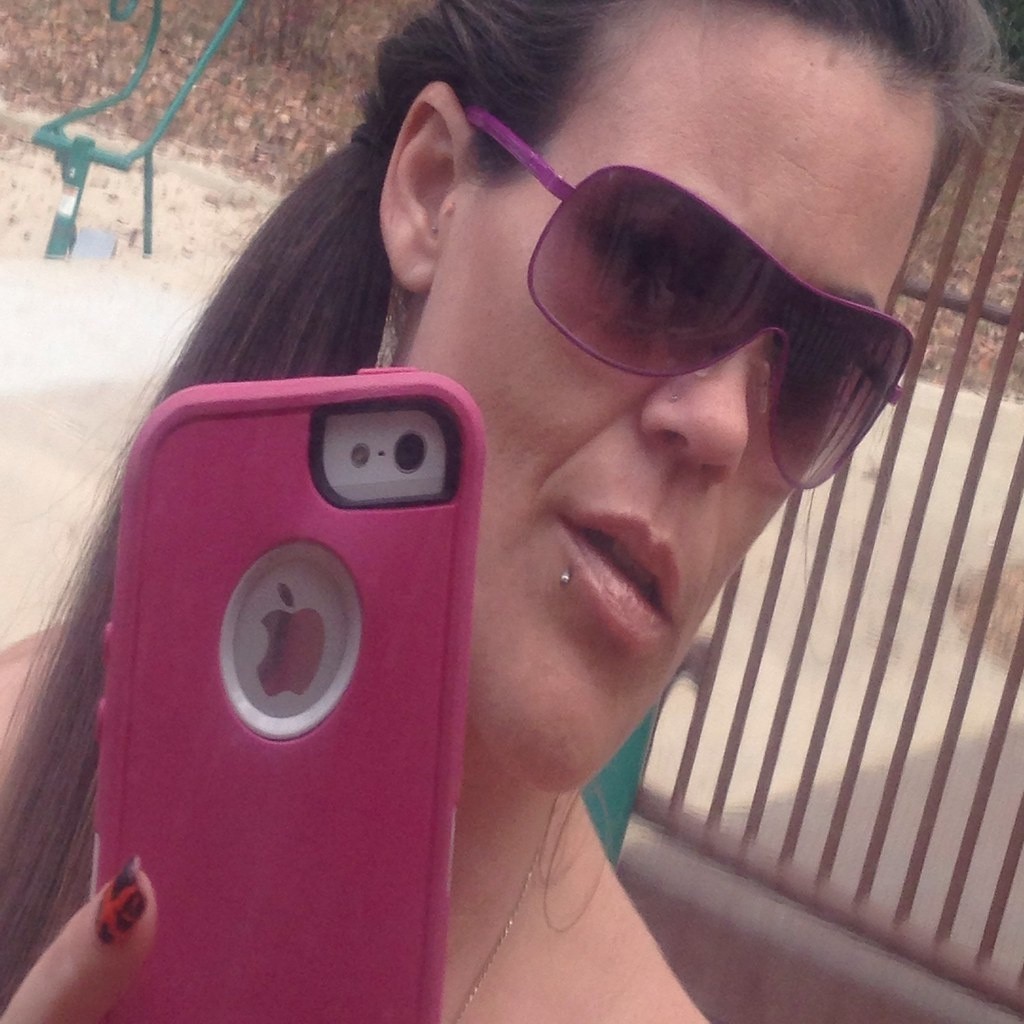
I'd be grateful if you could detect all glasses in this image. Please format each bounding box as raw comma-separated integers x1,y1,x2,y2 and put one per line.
460,109,918,490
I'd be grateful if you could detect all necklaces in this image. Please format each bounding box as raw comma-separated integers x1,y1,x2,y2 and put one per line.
454,793,563,1023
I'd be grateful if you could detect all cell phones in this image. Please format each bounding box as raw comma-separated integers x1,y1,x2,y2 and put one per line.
84,365,487,1024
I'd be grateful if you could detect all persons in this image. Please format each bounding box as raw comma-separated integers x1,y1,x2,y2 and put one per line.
0,0,1024,1024
